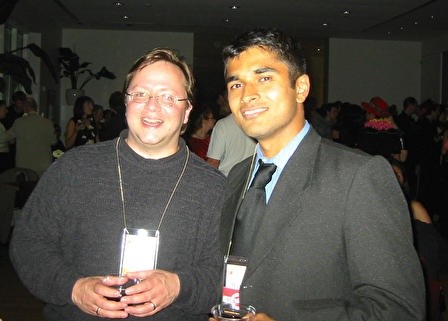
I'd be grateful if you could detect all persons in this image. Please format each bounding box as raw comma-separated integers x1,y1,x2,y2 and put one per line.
9,45,227,321
0,86,448,292
209,26,427,321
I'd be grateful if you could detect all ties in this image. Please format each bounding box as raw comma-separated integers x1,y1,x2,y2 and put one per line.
226,159,277,265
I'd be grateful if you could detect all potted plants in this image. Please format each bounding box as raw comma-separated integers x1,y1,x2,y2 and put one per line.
57,46,117,105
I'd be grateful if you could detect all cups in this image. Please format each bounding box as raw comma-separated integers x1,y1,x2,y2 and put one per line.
103,272,141,302
211,302,256,321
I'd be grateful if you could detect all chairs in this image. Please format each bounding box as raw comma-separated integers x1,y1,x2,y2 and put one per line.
0,167,39,245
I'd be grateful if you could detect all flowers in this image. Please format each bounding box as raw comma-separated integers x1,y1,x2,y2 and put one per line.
53,149,64,159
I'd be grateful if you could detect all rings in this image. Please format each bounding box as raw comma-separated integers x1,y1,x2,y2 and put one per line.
149,301,156,311
95,308,102,315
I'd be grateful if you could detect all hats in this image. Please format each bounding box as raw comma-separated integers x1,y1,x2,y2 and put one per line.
361,97,390,118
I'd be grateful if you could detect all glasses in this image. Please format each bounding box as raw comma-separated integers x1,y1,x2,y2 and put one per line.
124,92,188,106
